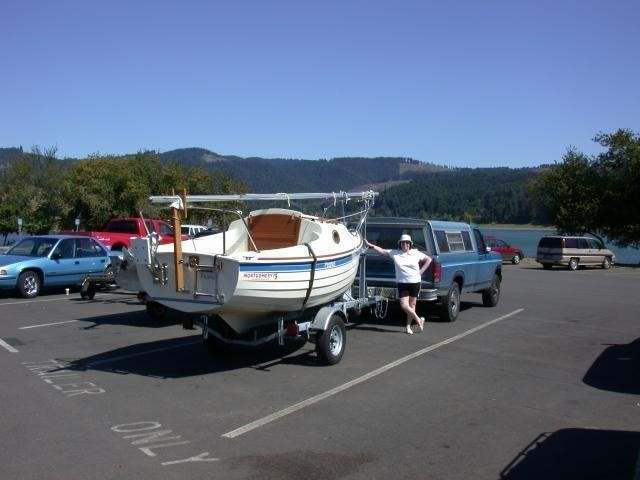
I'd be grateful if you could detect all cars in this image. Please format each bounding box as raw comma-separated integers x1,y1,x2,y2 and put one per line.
483,236,525,266
1,233,118,299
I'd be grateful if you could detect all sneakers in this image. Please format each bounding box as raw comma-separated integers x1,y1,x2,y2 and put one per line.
417,317,425,334
405,326,413,334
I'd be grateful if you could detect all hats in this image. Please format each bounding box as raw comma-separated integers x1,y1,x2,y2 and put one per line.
398,234,413,243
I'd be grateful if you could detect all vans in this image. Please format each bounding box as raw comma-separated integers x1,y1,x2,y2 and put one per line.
172,224,207,236
536,236,617,270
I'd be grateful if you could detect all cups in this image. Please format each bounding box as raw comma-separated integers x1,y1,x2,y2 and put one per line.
65,288,70,295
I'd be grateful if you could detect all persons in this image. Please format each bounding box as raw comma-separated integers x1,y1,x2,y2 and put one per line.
363,233,433,335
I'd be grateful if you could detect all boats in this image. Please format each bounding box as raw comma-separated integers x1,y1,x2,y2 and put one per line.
128,191,383,335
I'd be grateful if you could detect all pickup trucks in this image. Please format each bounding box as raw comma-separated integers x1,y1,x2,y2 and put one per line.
58,217,190,256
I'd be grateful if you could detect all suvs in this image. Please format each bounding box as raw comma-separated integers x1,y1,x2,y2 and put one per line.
344,217,504,321
